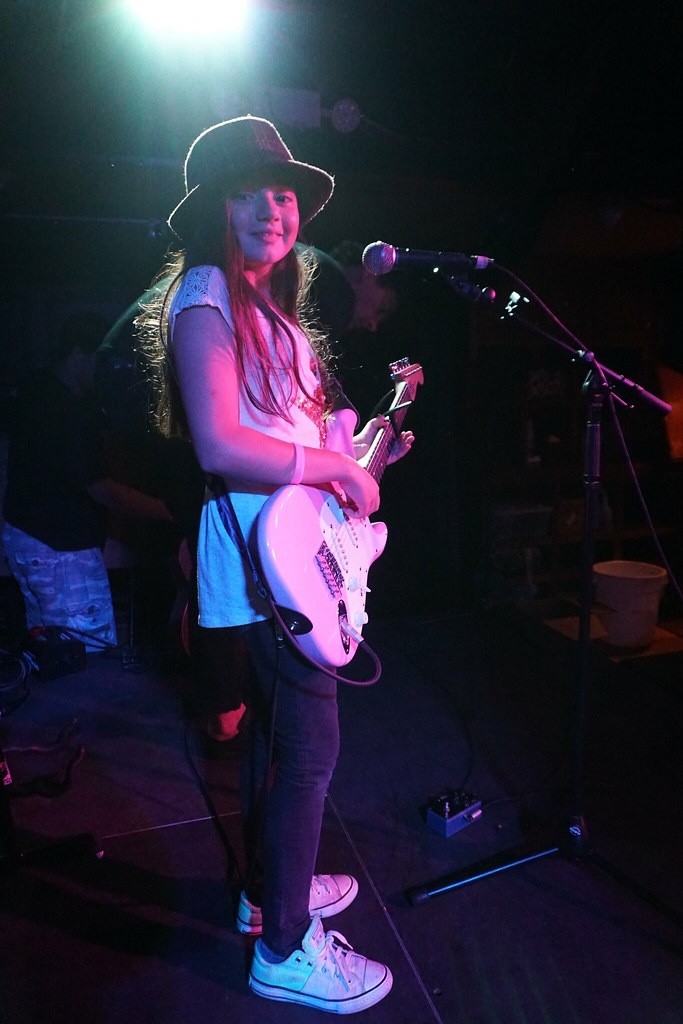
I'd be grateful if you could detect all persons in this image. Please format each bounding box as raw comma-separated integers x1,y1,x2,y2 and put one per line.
95,239,407,740
4,309,179,656
0,723,85,799
158,117,414,1014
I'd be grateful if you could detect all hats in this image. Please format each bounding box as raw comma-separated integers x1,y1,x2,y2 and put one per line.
168,114,336,246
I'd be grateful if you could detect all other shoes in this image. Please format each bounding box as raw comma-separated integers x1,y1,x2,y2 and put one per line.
211,703,248,741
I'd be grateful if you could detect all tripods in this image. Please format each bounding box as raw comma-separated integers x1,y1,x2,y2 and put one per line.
405,272,683,933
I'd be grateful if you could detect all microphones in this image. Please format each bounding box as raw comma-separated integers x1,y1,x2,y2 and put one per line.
362,241,492,275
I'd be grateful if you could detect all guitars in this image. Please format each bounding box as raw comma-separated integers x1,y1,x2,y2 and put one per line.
251,356,423,670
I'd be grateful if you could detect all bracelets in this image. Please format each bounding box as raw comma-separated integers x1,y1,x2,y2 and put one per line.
290,442,305,484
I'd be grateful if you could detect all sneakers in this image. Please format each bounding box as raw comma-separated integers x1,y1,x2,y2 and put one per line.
248,914,393,1015
236,873,359,934
